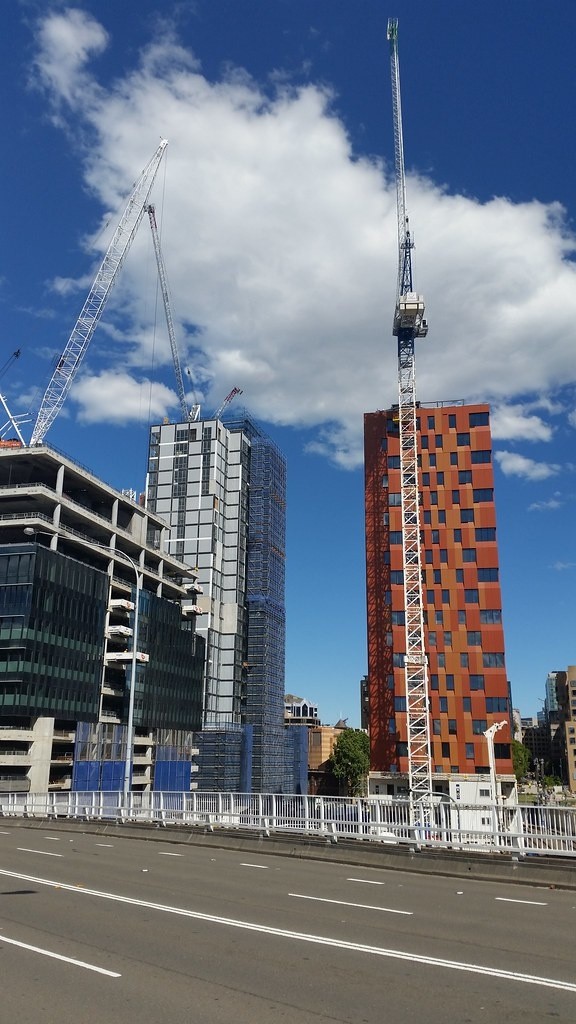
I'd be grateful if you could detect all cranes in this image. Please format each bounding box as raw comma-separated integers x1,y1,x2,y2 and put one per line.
384,17,430,844
144,203,243,422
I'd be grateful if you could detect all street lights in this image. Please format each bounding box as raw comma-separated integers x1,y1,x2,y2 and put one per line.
29,138,170,448
24,528,139,810
